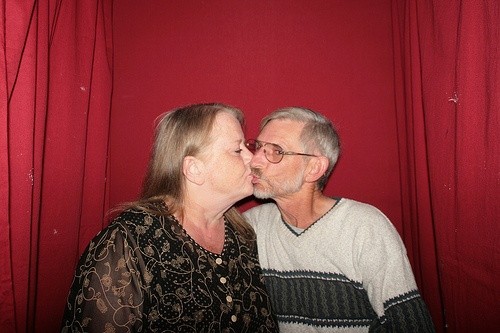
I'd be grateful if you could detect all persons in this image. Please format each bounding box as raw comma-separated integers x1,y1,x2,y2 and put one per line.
60,100,280,332
237,105,436,332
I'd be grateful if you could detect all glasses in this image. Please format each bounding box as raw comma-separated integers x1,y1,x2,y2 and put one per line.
245,138,320,165
